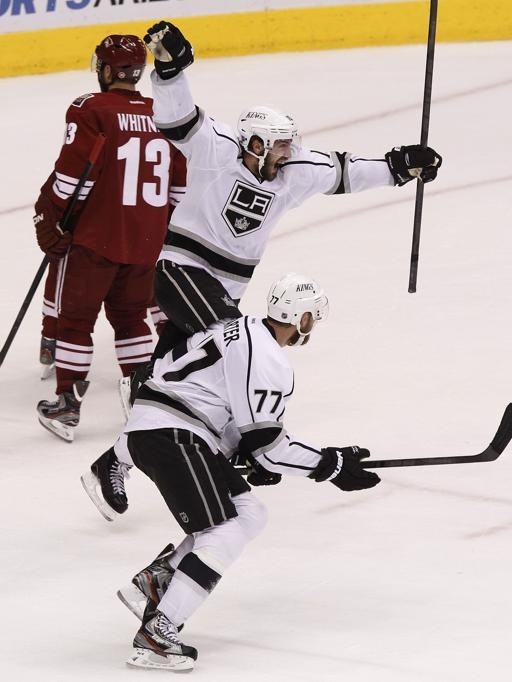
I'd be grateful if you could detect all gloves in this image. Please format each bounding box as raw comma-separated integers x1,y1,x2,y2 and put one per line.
143,20,195,81
246,457,282,487
308,442,382,493
32,212,73,262
384,145,442,188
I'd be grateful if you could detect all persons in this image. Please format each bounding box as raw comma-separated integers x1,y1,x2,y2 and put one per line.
31,34,187,428
90,21,442,513
122,272,381,660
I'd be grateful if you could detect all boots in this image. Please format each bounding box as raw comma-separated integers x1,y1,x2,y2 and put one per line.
134,598,197,661
128,366,151,404
81,444,134,523
37,381,89,427
116,542,184,633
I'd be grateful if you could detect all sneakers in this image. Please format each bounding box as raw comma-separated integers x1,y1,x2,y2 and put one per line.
39,336,56,365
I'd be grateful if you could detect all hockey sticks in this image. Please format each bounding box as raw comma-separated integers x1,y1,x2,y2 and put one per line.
234,403,511,476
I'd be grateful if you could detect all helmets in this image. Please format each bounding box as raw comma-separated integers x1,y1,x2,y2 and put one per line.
266,273,329,337
236,107,299,184
91,34,147,87
